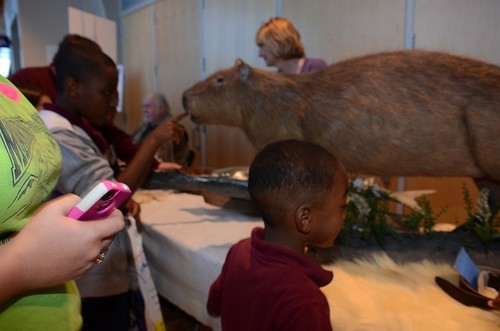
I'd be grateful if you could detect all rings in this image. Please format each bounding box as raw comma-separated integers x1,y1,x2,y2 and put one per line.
97,251,106,265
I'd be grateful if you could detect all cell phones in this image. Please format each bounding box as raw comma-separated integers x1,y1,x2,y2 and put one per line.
66,179,131,221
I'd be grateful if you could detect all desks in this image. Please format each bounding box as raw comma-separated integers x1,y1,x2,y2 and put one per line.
134,189,500,331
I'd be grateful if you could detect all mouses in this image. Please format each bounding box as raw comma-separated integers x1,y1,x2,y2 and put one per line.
181,50,500,233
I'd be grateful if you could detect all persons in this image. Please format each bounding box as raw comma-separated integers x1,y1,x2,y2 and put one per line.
255,17,327,74
207,139,349,331
0,33,190,331
